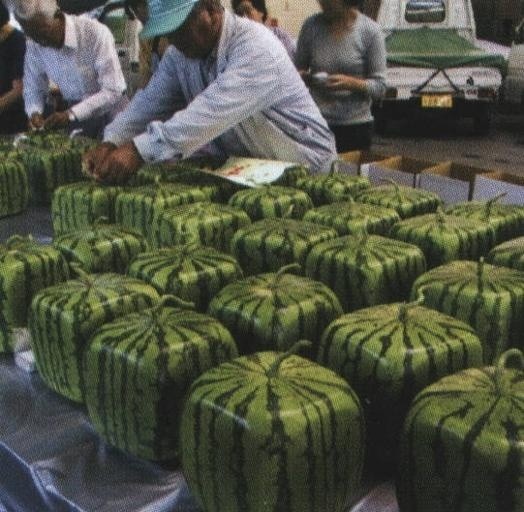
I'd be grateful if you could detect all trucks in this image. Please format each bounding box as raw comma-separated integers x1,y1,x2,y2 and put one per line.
376,0,503,122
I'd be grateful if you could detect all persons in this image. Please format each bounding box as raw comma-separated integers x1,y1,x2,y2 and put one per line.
85,0,339,185
294,0,388,154
229,0,298,64
1,0,171,138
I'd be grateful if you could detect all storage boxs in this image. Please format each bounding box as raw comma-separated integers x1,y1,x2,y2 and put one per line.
336,149,524,208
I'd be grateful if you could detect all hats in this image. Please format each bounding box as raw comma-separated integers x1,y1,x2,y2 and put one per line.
138,1,197,41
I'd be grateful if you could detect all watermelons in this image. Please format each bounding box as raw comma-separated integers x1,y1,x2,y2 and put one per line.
0,126,102,218
1,151,524,512
